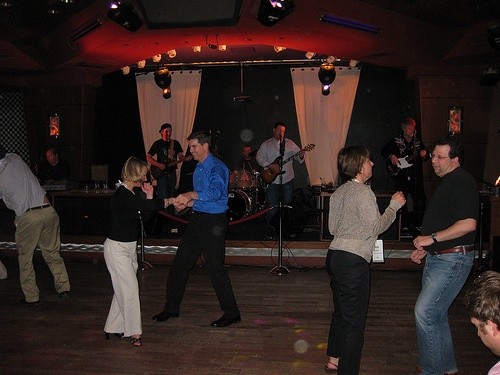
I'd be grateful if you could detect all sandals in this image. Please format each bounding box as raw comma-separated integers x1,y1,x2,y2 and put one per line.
324,360,338,373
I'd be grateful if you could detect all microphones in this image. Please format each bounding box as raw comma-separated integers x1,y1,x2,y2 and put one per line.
165,130,168,139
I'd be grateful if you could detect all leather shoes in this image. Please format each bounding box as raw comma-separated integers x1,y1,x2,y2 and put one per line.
211,310,241,328
152,311,176,321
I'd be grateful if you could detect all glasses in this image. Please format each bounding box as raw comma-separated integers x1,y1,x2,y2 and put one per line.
430,152,451,159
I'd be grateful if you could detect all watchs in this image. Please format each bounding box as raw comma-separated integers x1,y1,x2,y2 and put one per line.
432,232,438,242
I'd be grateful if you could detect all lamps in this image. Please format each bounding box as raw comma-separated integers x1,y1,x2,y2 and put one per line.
48,114,62,141
119,43,360,102
446,104,464,134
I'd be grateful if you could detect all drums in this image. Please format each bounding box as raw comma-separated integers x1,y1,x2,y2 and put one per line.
226,169,257,223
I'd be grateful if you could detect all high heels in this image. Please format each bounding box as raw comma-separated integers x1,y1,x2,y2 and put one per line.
105,331,123,340
130,333,144,346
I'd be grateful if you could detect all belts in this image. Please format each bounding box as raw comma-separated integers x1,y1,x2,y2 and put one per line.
429,246,476,256
25,204,50,211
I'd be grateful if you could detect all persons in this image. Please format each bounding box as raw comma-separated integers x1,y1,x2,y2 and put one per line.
0,145,71,309
323,143,407,375
146,122,194,205
464,270,500,375
381,116,433,241
41,147,72,181
150,131,243,328
104,156,157,349
234,143,258,171
256,122,307,242
407,138,481,374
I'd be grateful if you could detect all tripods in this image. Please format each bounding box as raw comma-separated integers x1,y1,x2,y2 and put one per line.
136,208,152,269
269,131,292,274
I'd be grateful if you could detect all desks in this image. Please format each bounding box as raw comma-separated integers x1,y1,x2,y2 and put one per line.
46,187,116,219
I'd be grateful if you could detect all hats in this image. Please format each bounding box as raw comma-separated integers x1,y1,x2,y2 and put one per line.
158,123,171,132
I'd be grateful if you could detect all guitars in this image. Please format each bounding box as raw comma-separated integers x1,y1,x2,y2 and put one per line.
387,140,433,178
259,144,316,184
150,155,193,179
